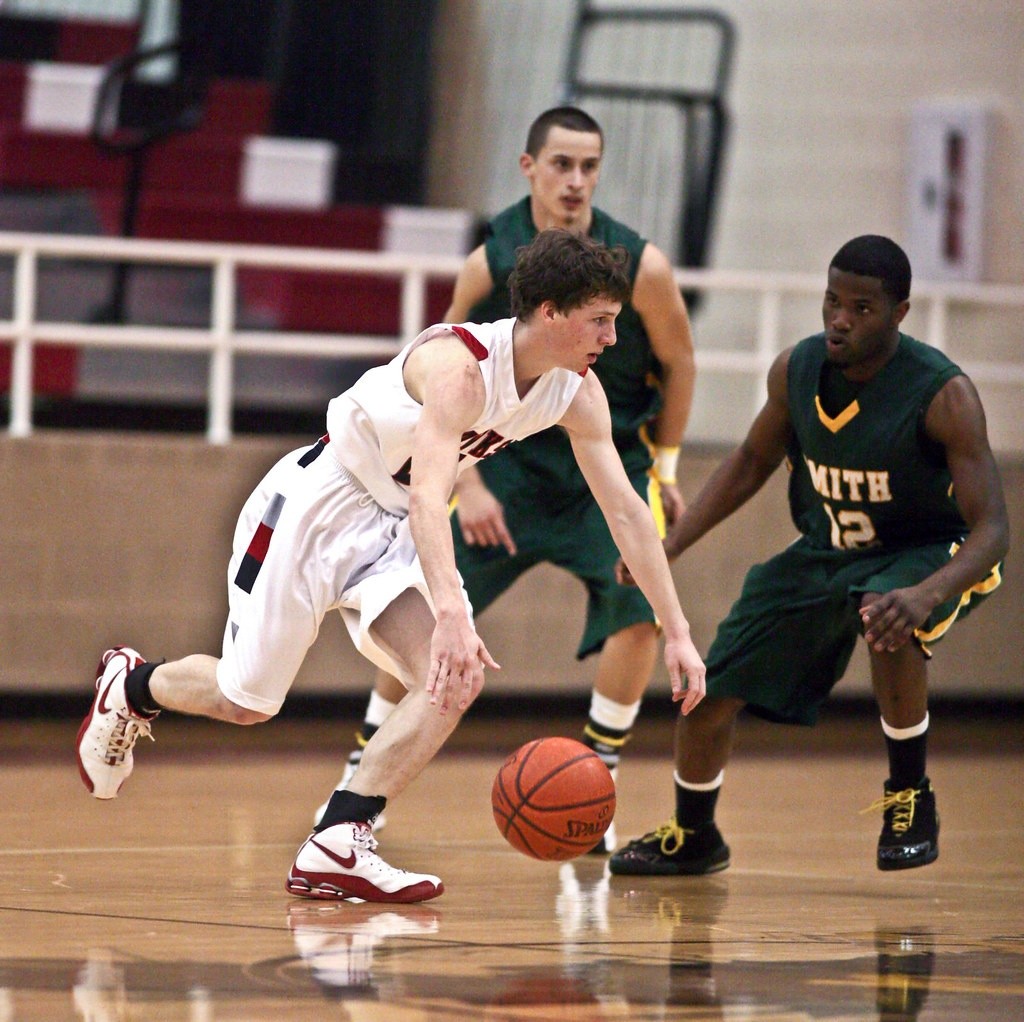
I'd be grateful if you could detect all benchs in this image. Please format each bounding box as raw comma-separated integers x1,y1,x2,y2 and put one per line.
0,12,482,355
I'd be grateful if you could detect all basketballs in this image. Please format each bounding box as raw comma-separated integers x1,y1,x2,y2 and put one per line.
491,736,617,863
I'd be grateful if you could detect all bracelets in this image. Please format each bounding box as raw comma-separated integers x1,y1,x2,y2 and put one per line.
653,445,681,485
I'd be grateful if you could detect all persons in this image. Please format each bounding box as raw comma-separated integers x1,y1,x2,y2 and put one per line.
608,234,1008,874
74,228,708,905
73,856,939,1022
315,102,697,855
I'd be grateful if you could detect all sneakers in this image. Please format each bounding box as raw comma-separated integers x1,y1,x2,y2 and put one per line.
591,819,617,854
74,644,162,800
609,807,731,874
859,777,941,870
284,821,445,903
313,730,387,833
